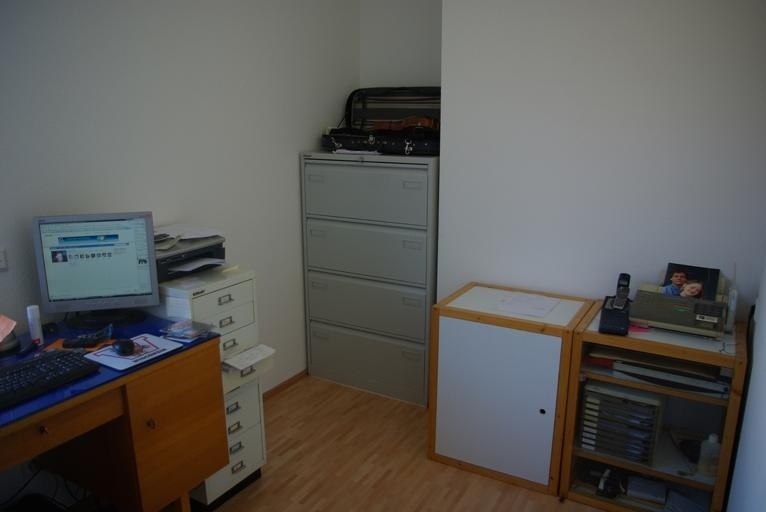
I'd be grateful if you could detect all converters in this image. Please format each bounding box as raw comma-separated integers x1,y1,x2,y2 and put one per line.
42,322,58,338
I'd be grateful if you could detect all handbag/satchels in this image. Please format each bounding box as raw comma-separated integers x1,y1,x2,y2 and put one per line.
322,87,440,156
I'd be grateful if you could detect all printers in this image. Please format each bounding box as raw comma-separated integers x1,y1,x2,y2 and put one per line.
154,235,225,283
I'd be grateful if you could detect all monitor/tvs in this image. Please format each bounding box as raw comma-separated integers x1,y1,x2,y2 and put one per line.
32,211,161,329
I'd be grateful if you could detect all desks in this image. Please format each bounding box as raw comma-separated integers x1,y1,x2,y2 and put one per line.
0,309,231,512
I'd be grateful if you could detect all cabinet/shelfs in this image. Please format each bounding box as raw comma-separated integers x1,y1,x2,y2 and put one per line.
143,264,276,512
300,150,439,409
426,281,595,496
558,299,748,512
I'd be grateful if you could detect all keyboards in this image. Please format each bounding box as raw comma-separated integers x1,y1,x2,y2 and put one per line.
0,350,101,411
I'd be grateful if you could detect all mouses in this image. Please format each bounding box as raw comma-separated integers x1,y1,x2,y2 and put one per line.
112,339,134,357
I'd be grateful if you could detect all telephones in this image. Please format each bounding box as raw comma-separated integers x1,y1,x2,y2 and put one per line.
598,272,631,336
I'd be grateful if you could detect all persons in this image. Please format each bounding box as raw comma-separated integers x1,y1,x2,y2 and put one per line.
661,270,687,296
54,252,64,262
677,280,704,298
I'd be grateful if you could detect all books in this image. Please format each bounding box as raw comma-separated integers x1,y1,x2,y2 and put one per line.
582,345,732,512
